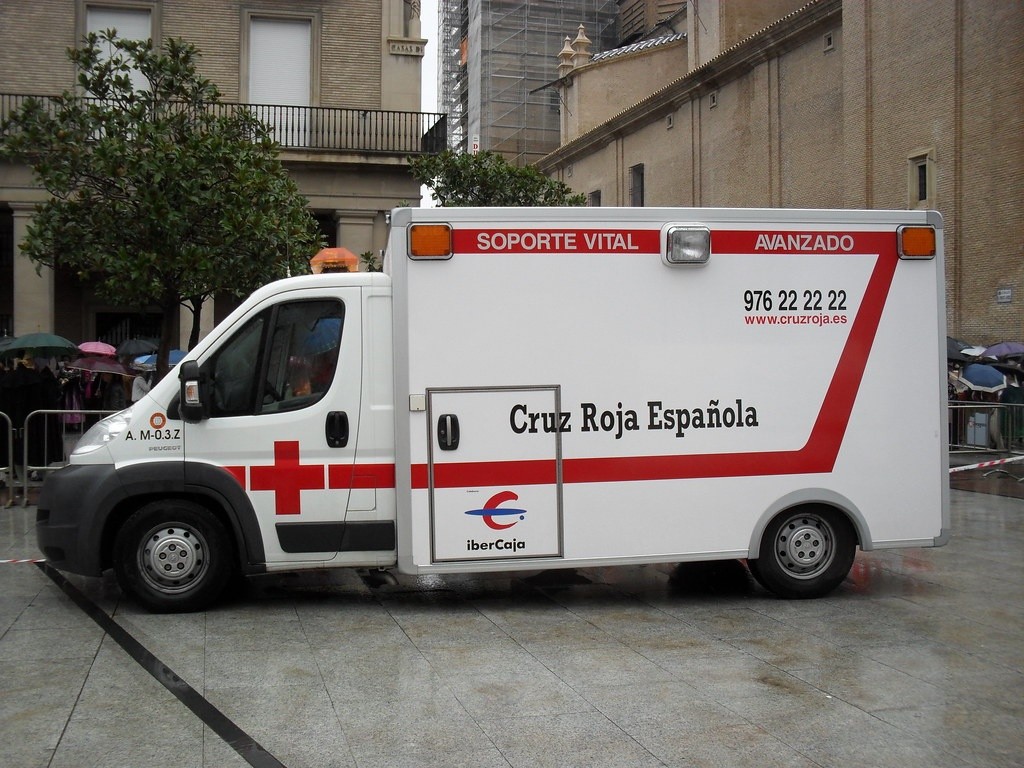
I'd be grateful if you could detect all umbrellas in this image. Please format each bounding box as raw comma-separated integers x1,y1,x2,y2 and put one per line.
947,336,1024,394
0,332,80,359
65,339,190,373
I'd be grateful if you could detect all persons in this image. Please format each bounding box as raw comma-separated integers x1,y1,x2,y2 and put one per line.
0,361,153,432
947,373,1024,450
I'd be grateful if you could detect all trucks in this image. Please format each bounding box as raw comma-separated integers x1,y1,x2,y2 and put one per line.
33,204,952,615
